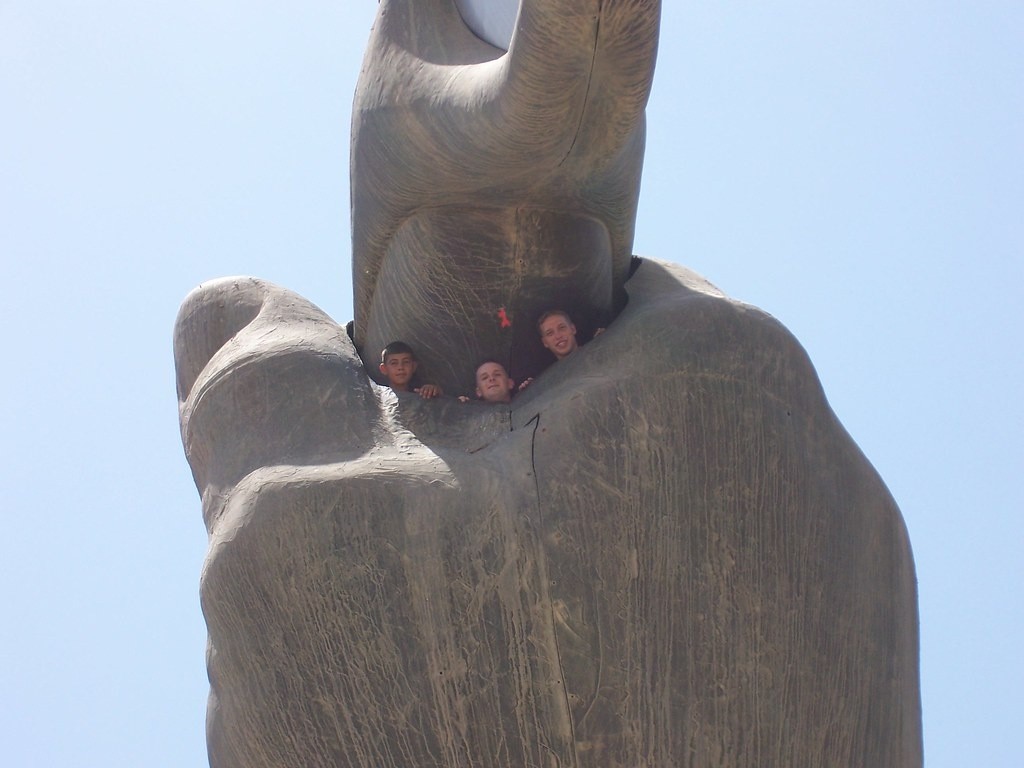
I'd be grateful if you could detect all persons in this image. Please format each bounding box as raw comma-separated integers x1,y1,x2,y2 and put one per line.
459,360,533,402
540,310,606,359
379,342,443,399
